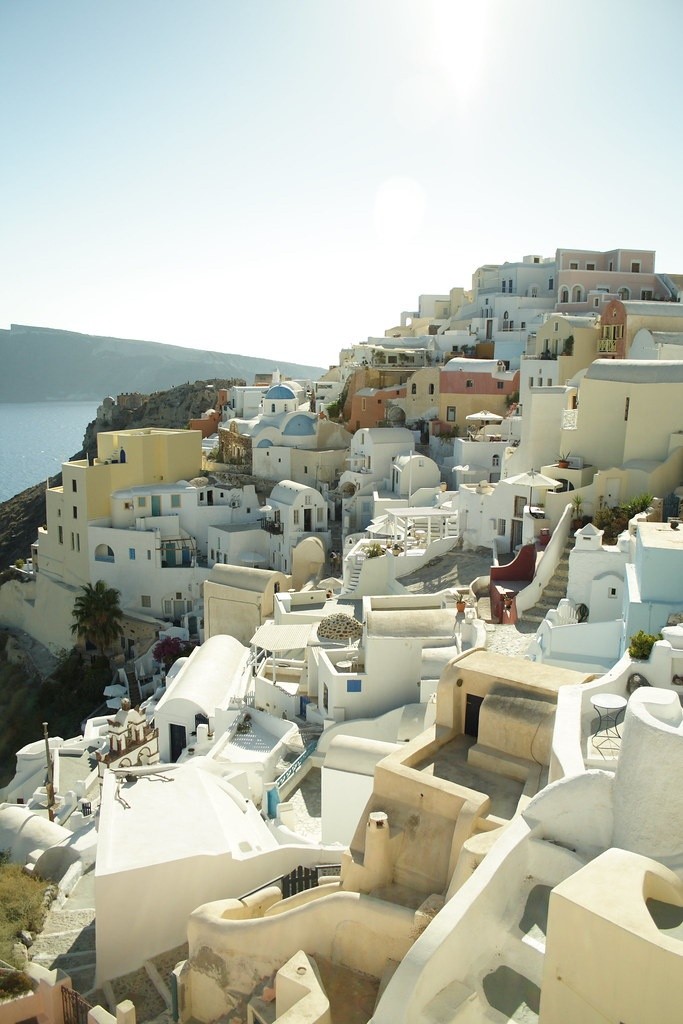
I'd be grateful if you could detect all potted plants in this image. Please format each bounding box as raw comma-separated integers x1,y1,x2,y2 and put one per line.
555,452,571,468
367,542,400,557
326,591,331,598
453,593,466,612
571,494,585,528
504,595,511,607
188,748,194,756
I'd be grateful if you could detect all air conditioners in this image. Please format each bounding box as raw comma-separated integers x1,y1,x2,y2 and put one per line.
567,456,583,469
230,501,238,507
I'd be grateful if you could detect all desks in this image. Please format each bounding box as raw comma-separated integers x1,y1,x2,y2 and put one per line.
336,660,353,672
590,693,629,740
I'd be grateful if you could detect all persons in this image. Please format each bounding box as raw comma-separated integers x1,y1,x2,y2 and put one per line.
119,446,126,463
282,709,288,720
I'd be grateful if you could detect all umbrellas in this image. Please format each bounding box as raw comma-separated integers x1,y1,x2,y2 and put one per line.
497,467,564,512
466,409,503,441
344,452,366,472
258,504,280,526
364,513,416,539
